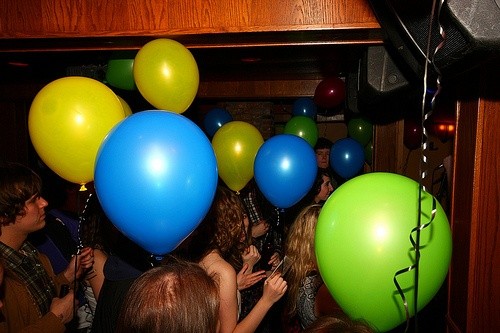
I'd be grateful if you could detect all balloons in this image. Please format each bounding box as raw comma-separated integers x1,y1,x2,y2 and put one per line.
313,170,453,333
403,123,427,150
27,35,372,260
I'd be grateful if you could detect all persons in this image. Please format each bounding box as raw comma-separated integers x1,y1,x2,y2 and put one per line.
0,137,381,333
443,155,453,194
